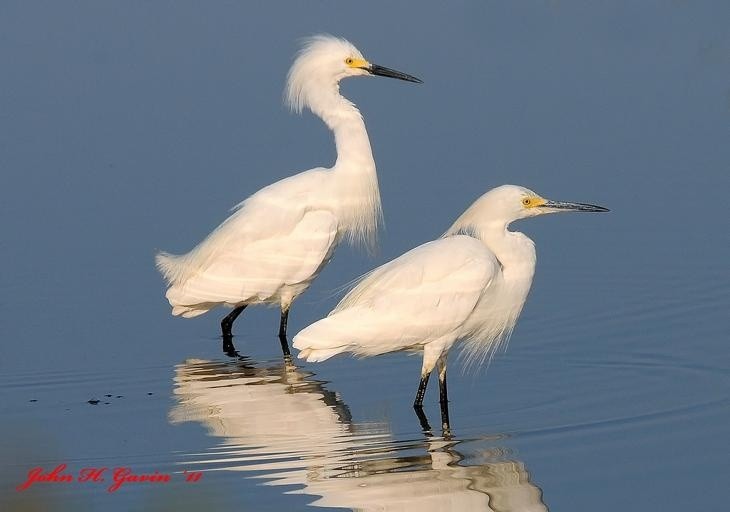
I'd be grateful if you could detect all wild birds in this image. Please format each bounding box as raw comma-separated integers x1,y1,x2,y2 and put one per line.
154,32,424,336
244,401,550,512
166,333,510,489
291,183,612,406
